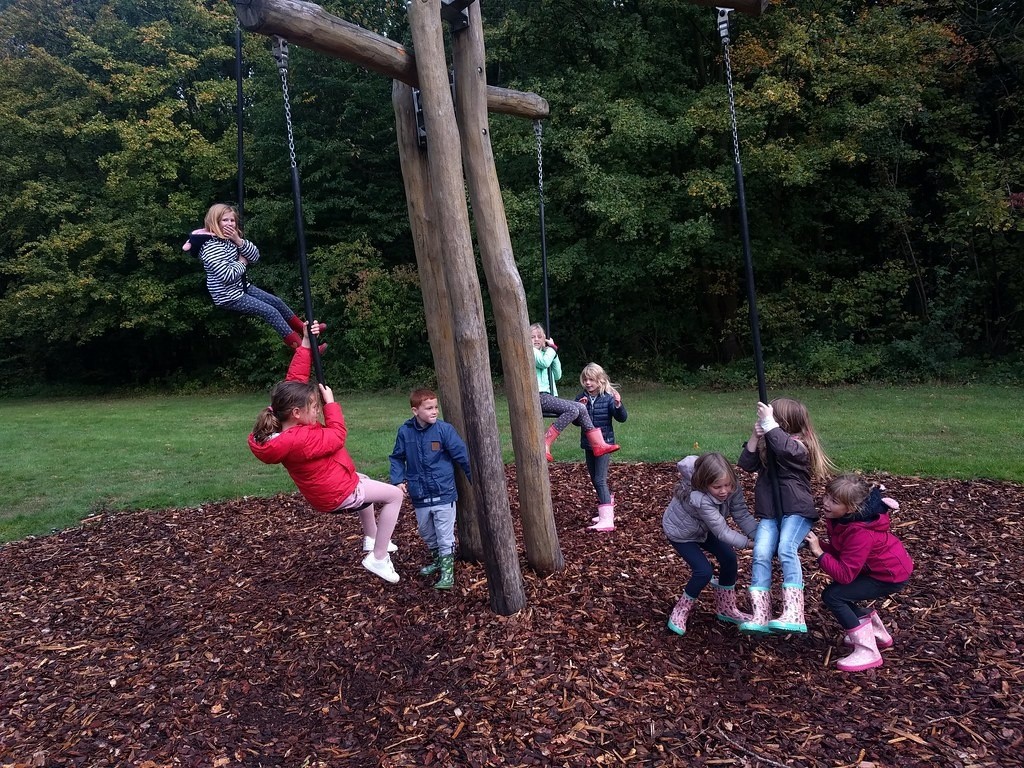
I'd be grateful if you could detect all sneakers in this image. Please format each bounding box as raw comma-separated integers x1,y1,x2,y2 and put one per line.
361,551,400,583
363,535,399,552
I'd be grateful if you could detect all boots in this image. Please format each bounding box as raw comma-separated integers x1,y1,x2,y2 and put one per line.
420,549,440,576
768,583,808,634
288,315,327,336
836,618,883,672
585,428,620,457
283,331,328,363
710,579,754,625
586,503,615,532
843,609,893,648
434,554,454,590
544,424,560,462
667,592,696,635
739,586,773,636
592,495,615,523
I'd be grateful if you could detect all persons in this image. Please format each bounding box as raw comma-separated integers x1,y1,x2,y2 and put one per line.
388,389,473,590
530,322,621,463
661,451,758,635
247,320,404,584
572,362,627,532
804,474,915,672
182,204,327,361
739,398,841,633
